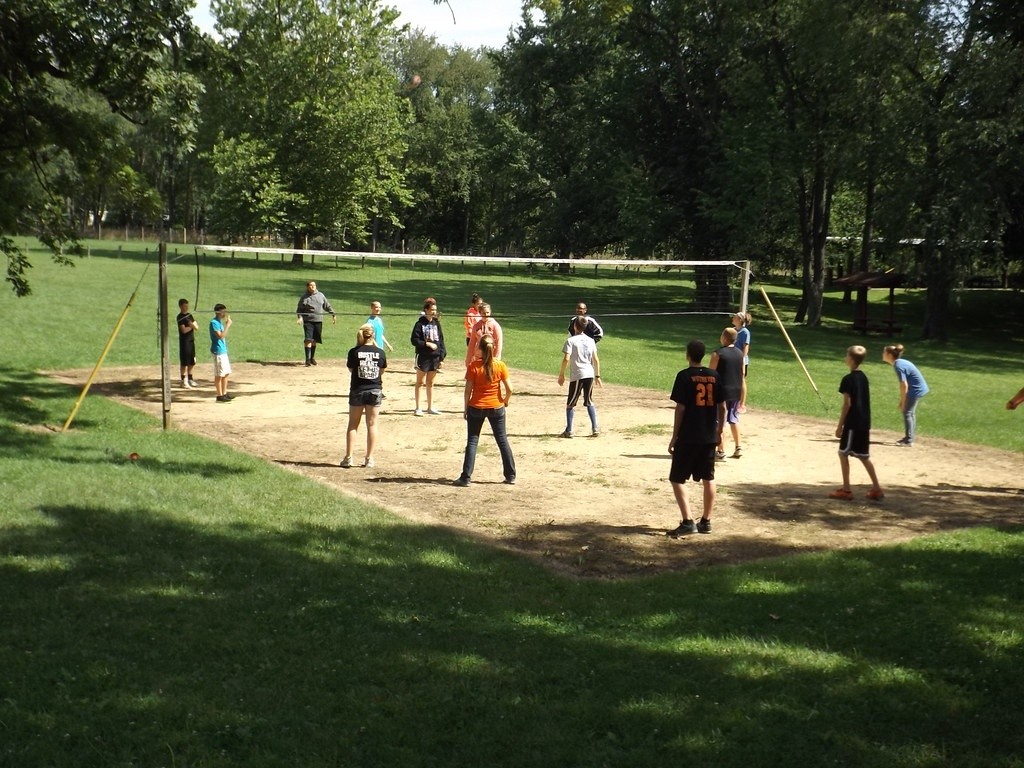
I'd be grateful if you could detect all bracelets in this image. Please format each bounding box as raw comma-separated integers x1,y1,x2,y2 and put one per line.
595,375,601,378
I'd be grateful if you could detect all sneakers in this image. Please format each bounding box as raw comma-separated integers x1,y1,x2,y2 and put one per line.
695,521,712,533
666,520,698,537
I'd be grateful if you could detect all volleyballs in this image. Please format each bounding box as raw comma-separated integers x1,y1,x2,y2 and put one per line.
407,75,421,88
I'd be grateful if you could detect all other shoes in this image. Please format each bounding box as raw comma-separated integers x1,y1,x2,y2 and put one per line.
189,379,198,386
715,451,727,462
592,426,600,437
559,431,576,438
829,489,854,501
734,448,743,457
738,405,747,413
364,456,375,468
504,478,515,483
428,409,441,414
340,456,353,467
216,393,237,403
866,489,884,500
305,358,317,366
895,438,914,447
453,477,470,487
180,379,192,388
414,408,423,416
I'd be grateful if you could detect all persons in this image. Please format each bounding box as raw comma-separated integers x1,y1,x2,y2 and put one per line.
176,298,200,389
296,280,336,367
209,303,236,403
410,297,447,416
709,327,745,462
465,302,503,369
667,339,727,536
339,323,387,468
558,314,603,438
731,312,752,414
882,344,930,447
465,292,484,347
453,333,517,487
567,302,603,344
1006,387,1024,410
827,345,885,501
365,300,394,400
